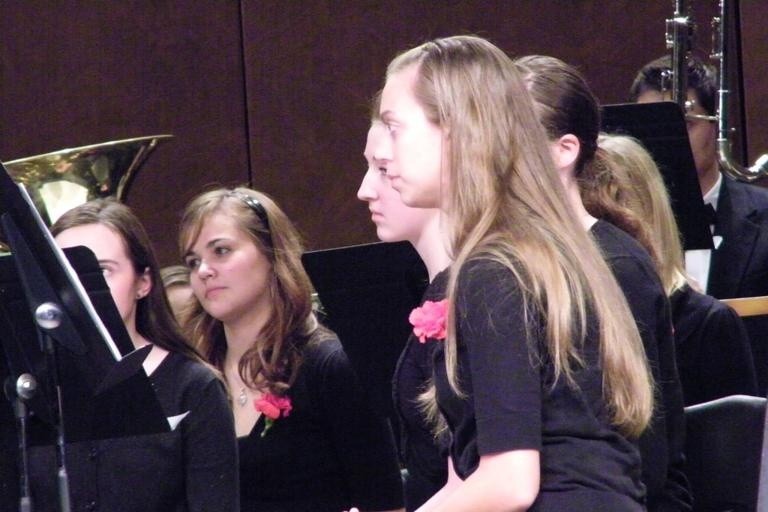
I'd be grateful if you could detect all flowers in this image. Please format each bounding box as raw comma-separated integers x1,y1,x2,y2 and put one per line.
254,392,292,437
409,299,448,344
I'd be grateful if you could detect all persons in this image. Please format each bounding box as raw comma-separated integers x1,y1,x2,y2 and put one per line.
0,197,244,510
354,88,463,510
633,52,768,303
374,34,649,512
510,53,695,510
576,128,761,408
154,263,210,357
176,185,407,511
